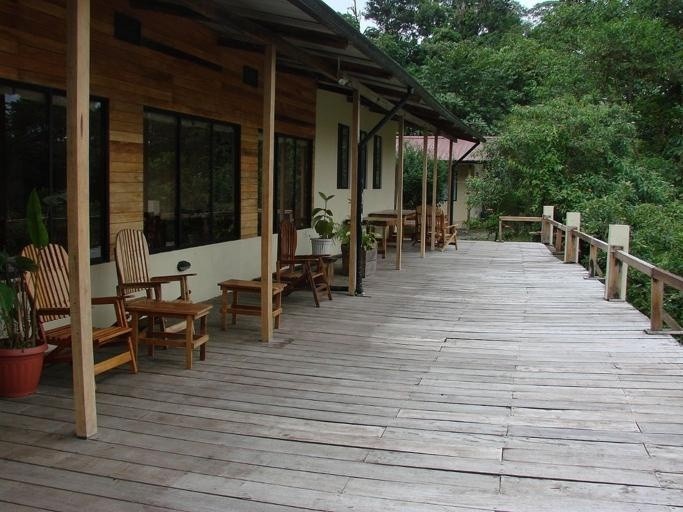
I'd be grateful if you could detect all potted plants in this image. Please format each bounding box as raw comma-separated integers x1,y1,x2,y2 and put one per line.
310,191,340,256
0,189,49,399
337,219,376,278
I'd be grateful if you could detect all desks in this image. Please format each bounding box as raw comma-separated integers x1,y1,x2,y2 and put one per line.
363,209,415,260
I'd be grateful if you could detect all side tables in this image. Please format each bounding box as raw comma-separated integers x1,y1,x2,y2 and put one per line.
217,279,287,331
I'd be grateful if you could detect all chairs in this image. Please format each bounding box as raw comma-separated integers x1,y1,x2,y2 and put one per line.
275,219,336,308
416,204,460,253
21,228,214,391
